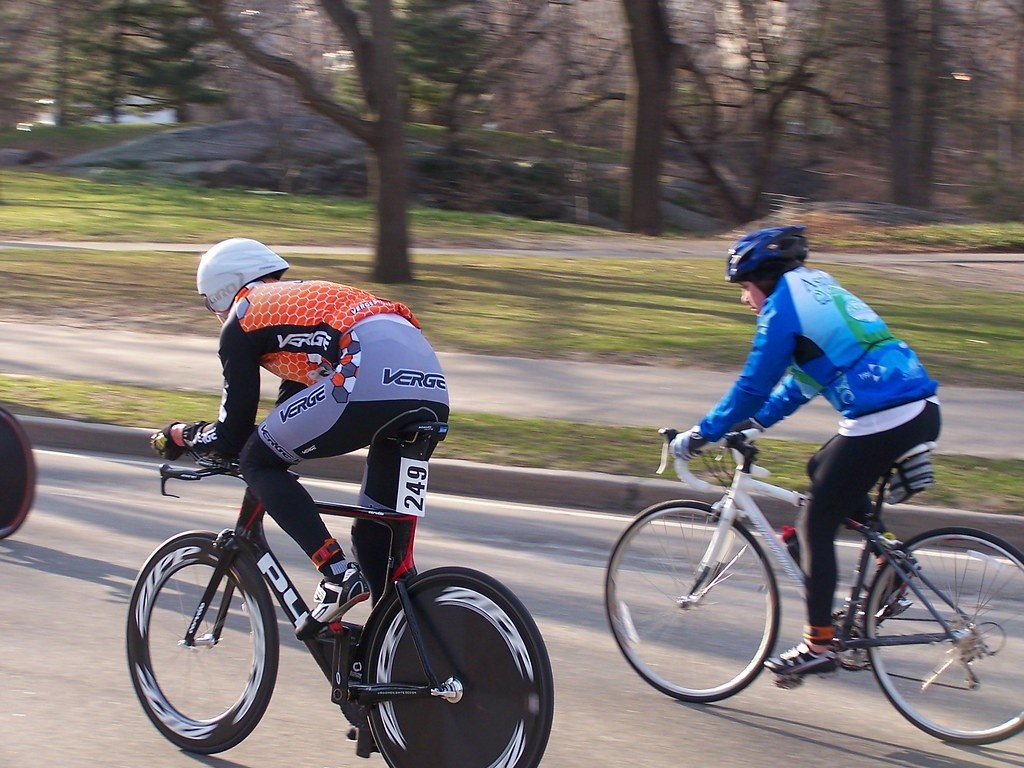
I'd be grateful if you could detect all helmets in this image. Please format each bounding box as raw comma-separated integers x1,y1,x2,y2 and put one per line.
195,238,288,312
723,221,809,283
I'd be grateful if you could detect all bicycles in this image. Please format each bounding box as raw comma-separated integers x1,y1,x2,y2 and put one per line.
602,426,1024,747
123,422,555,768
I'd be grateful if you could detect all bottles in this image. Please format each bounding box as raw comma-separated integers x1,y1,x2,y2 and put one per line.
782,524,799,566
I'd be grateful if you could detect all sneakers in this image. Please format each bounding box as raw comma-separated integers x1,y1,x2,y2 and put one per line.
293,559,370,641
764,643,840,680
863,554,922,588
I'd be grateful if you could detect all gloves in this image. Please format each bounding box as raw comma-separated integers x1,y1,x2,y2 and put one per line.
669,425,707,462
150,418,182,460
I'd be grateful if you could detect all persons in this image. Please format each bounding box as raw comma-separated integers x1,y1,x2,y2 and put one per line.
152,239,449,752
672,225,940,676
